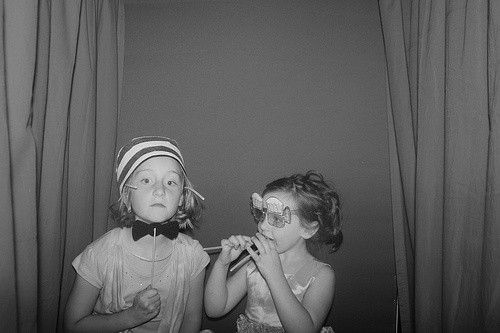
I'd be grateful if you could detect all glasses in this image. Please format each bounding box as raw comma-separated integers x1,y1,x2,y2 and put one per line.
250,193,304,228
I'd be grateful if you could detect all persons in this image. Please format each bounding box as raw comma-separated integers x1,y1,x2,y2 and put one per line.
204,169,344,332
62,136,210,333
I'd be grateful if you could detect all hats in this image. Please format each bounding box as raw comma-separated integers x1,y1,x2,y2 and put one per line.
116,136,187,196
